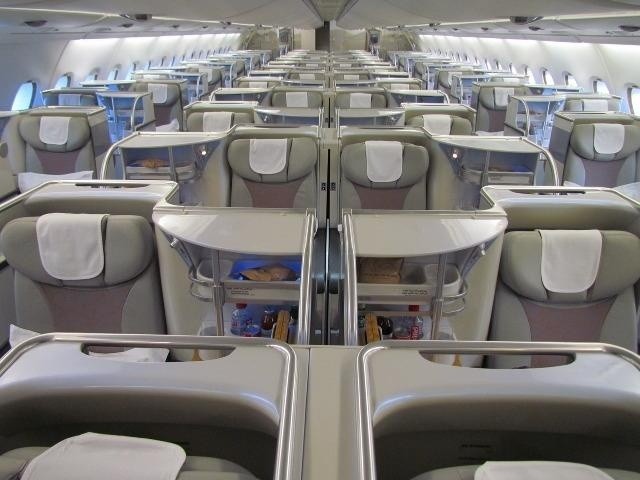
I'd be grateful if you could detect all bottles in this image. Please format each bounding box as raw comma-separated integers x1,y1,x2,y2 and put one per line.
359,302,424,343
230,303,297,344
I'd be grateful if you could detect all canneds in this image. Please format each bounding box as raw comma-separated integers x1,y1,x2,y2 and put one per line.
242,325,262,337
393,327,412,340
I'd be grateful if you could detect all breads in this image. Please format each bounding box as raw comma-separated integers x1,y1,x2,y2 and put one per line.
273,309,291,342
365,313,381,343
